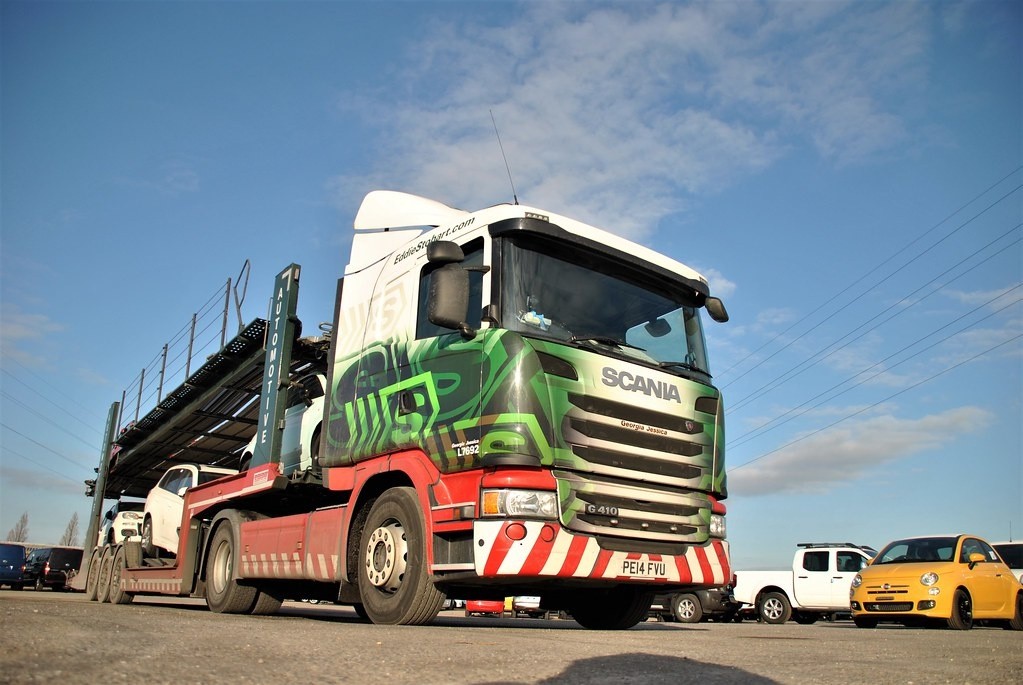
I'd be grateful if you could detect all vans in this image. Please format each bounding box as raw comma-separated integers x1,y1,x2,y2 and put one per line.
0,541,84,592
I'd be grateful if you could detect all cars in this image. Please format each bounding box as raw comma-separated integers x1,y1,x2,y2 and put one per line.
849,534,1023,630
442,587,740,623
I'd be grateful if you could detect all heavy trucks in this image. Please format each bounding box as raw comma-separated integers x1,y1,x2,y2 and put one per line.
84,190,734,631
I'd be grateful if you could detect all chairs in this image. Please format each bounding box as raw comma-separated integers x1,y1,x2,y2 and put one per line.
844,558,856,569
916,544,940,560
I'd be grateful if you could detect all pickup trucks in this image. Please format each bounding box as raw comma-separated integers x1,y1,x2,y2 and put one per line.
734,542,893,624
